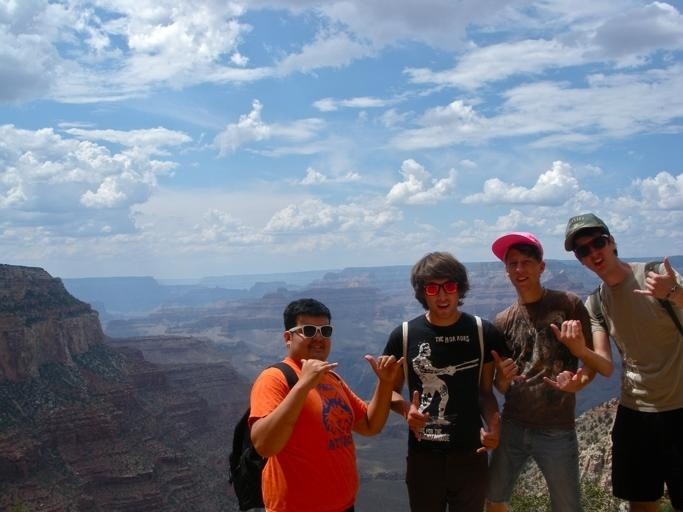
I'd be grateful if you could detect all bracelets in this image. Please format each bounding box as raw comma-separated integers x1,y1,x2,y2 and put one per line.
662,282,680,301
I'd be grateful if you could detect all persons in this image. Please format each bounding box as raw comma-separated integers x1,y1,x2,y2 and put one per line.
246,298,404,512
548,214,682,511
484,231,595,511
376,251,505,511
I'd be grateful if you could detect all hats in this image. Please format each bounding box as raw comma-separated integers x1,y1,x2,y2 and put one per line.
565,213,609,251
491,232,543,263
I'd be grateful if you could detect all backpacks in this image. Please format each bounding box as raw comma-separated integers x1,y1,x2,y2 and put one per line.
230,363,299,509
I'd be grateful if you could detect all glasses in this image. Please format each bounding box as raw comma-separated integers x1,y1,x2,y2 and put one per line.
289,325,333,338
574,234,608,257
425,282,457,295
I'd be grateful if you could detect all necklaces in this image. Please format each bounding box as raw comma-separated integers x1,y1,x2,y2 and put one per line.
516,286,547,338
